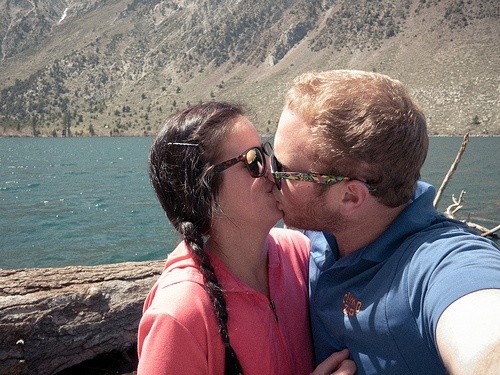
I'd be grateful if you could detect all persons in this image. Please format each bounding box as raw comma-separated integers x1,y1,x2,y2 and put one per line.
136,101,358,375
269,70,500,375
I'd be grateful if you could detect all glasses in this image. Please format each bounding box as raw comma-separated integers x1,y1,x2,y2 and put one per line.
210,141,274,178
270,154,376,193
